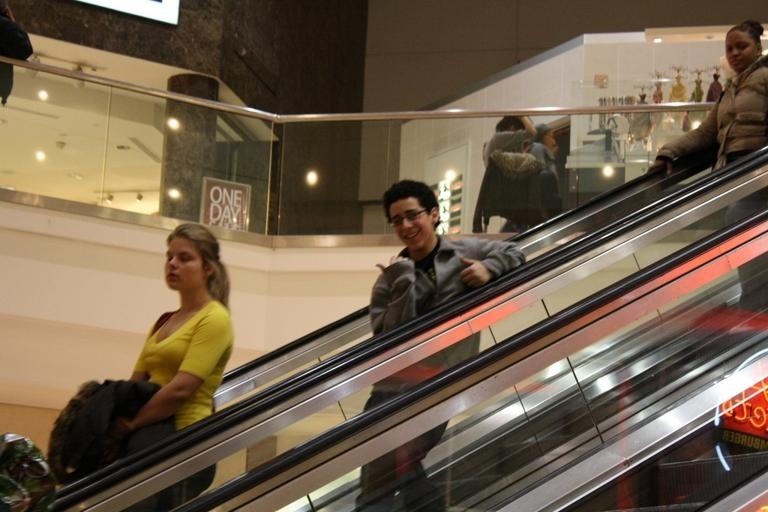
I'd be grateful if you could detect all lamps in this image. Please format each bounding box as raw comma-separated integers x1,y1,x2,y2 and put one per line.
25,49,87,89
642,21,768,44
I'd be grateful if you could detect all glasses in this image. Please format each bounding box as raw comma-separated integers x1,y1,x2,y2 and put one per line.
390,208,427,228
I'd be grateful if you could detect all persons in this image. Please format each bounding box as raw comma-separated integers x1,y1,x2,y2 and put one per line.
646,18,768,313
482,115,562,237
351,179,526,512
0,0,34,106
107,223,235,512
